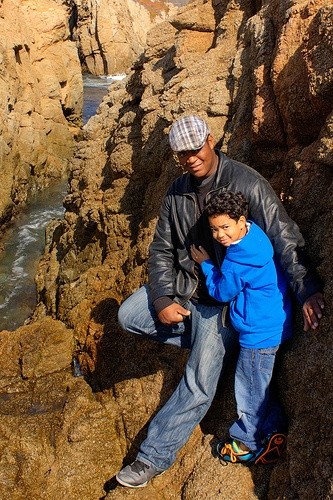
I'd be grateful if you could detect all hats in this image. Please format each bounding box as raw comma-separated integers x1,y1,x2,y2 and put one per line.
167,115,210,153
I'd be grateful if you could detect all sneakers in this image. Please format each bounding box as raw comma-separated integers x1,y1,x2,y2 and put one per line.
115,459,167,488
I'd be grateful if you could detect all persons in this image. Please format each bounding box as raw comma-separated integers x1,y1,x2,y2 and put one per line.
189,189,293,465
115,115,325,489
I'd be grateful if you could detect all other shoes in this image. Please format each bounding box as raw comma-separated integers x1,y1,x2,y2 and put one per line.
217,434,286,466
